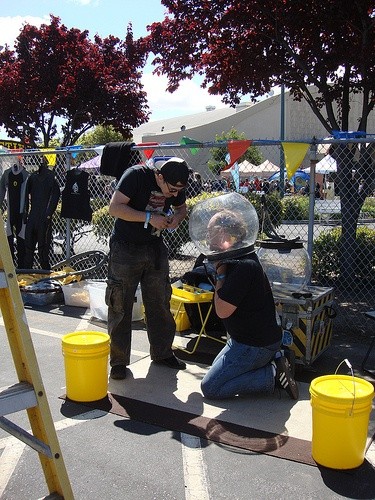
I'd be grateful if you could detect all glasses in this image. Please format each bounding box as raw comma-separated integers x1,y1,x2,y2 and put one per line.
164,178,184,193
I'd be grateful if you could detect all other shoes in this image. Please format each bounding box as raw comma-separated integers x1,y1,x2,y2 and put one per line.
154,355,186,369
110,364,127,379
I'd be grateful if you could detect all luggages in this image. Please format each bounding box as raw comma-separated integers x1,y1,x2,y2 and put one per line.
180,264,228,340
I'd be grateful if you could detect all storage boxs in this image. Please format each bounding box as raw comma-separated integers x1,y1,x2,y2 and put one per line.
269,281,334,366
60,279,214,332
21,281,63,305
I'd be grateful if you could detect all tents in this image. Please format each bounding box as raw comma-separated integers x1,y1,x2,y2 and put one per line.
303,153,356,199
70,155,102,171
220,159,280,182
268,168,311,193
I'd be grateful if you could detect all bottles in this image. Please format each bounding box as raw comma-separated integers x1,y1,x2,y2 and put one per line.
188,192,259,262
162,206,178,232
256,239,312,298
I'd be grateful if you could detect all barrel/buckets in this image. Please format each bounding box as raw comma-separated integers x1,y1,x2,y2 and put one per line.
309,358,375,469
61,331,110,401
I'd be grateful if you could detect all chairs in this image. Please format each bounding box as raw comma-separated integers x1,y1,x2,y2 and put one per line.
361,312,375,378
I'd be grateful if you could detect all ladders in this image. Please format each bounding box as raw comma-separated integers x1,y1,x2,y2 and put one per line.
0,207,75,500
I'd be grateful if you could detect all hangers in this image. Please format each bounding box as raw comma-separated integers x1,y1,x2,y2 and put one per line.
60,160,91,180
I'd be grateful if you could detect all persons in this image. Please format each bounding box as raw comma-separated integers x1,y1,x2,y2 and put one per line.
201,210,299,400
105,157,189,379
204,176,321,199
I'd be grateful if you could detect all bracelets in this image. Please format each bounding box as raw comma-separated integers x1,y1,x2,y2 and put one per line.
144,212,152,228
216,274,225,281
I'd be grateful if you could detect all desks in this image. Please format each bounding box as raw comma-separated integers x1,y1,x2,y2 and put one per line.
169,295,227,354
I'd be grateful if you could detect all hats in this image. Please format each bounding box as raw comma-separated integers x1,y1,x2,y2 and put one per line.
154,157,189,187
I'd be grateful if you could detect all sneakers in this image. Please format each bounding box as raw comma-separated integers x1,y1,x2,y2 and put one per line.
271,355,299,400
